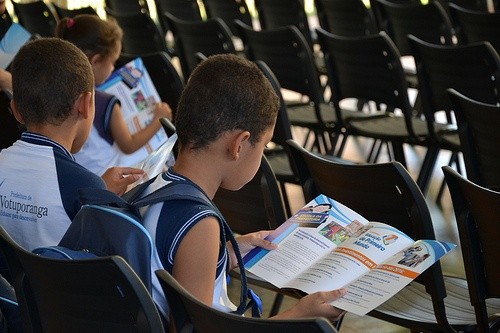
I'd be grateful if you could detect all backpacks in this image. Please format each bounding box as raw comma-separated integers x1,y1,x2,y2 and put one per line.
31,172,264,332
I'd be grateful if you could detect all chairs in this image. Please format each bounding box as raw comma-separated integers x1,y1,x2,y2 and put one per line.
0,0,500,333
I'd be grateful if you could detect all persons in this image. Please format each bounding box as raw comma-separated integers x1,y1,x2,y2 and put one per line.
0,0,347,332
